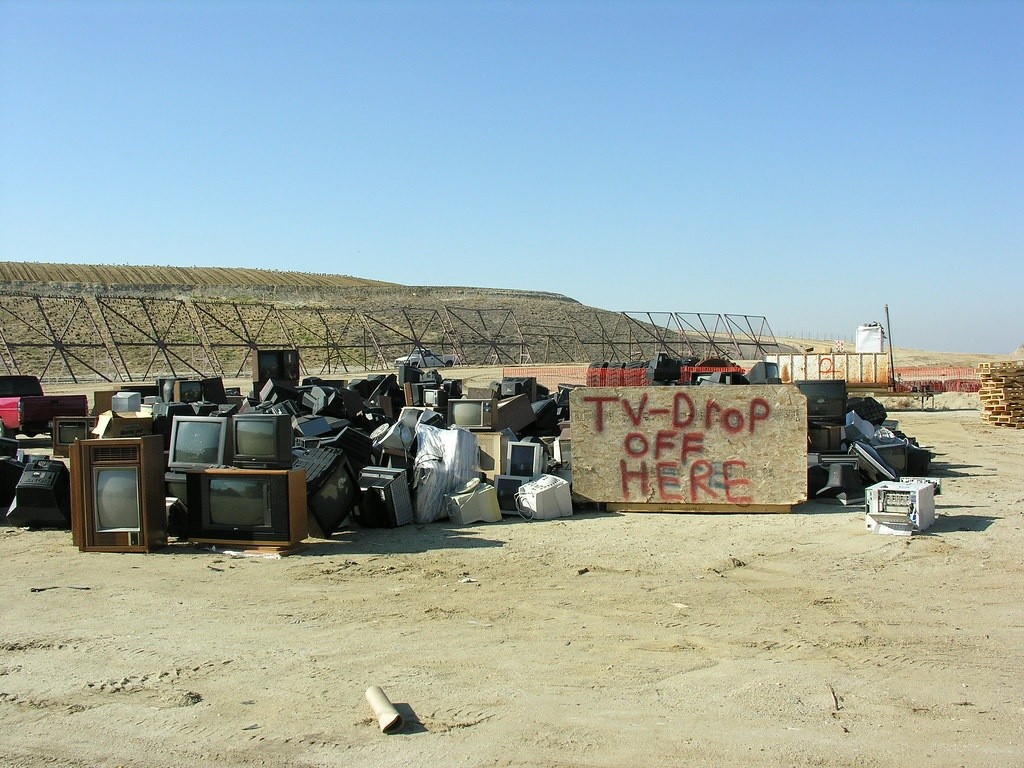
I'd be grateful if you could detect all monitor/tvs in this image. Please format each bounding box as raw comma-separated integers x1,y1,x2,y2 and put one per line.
689,361,941,537
0,348,586,553
